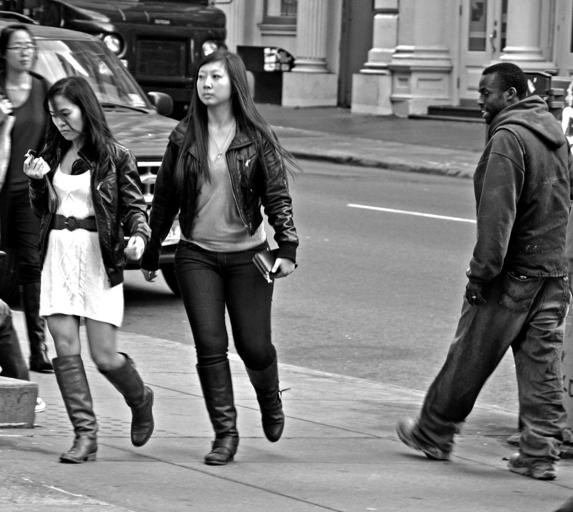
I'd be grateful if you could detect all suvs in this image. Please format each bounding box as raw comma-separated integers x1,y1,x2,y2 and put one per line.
0,20,182,296
1,1,228,117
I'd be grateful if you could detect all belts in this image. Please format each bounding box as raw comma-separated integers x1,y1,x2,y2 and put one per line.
48,214,96,233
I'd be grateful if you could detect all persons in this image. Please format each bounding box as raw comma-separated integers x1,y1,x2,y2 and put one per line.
395,62,573,479
23,76,154,463
1,298,46,412
1,25,53,374
562,81,573,157
141,49,299,466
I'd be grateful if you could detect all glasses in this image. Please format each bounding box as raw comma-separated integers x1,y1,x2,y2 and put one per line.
6,46,40,54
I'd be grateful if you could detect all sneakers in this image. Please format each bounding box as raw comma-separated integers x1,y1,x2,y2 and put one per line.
396,417,451,461
508,453,557,481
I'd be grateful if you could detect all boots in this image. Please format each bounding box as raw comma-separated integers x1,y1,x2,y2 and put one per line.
18,283,54,372
98,353,155,447
244,345,291,443
51,355,99,463
196,361,240,465
